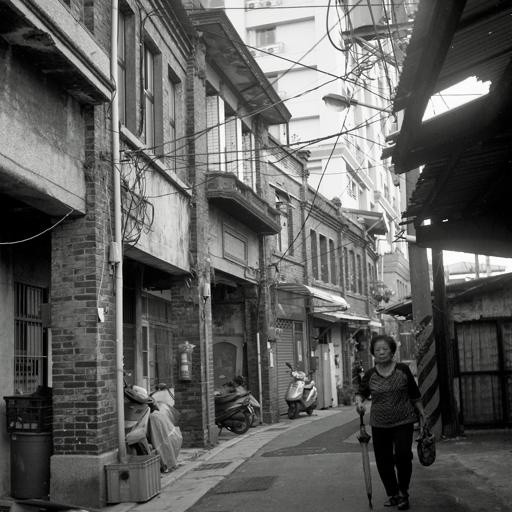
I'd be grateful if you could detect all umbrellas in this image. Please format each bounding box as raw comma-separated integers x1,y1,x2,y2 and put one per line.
355,405,373,508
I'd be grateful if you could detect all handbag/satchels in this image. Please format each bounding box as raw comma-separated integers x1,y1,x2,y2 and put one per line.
416,423,436,467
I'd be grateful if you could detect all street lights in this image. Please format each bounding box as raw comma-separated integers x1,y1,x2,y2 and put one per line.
321,93,464,438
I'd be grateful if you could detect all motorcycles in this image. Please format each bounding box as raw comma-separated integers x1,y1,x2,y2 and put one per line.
353,360,372,401
214,390,252,437
284,361,318,419
223,376,261,427
123,370,183,474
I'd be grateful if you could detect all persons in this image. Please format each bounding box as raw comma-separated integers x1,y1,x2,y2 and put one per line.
353,333,428,512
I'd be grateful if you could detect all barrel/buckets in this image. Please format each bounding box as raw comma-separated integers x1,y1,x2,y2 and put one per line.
10,432,52,499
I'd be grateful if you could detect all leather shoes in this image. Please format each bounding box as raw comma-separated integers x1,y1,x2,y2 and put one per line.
398,496,410,510
384,496,399,506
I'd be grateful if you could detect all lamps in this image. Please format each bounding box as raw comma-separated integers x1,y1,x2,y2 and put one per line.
108,240,122,275
200,282,212,303
